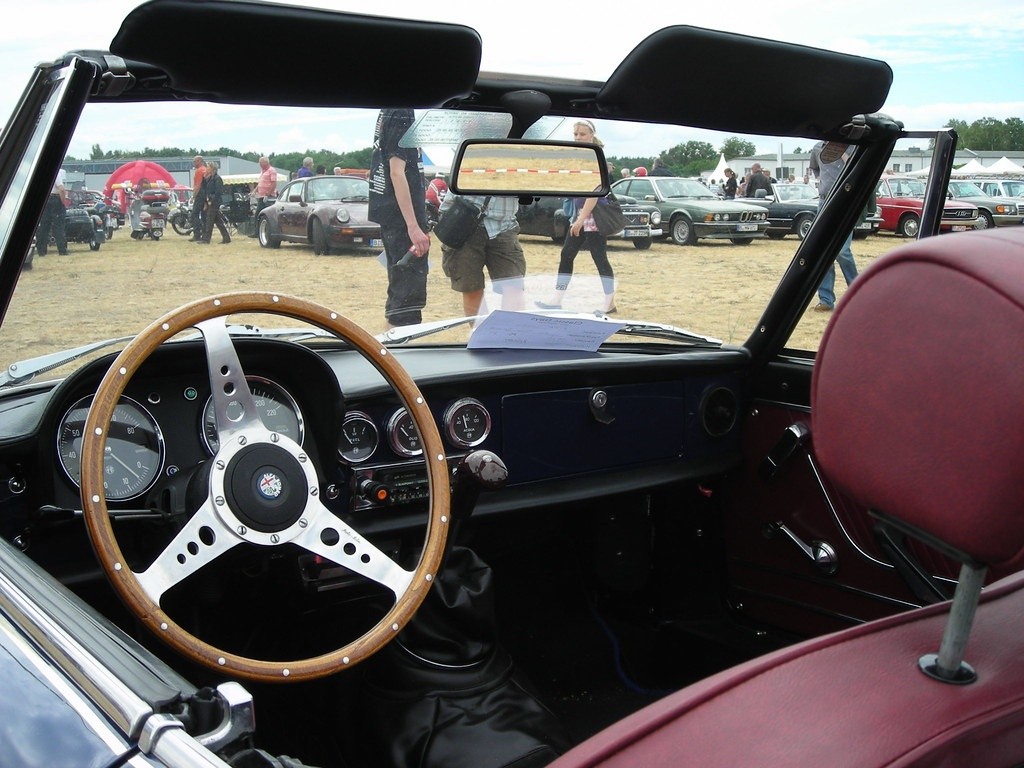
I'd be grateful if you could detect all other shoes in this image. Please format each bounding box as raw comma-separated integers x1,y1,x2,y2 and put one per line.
219,241,229,244
188,238,195,241
248,234,259,238
593,306,617,317
535,301,561,310
197,240,210,244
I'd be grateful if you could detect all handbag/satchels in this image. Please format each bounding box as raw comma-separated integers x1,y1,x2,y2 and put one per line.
433,197,486,250
592,193,626,237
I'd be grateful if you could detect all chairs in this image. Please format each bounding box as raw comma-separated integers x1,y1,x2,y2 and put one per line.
672,183,684,195
754,189,767,200
547,223,1024,768
993,189,1001,196
308,189,316,200
954,189,962,196
336,186,349,198
780,191,790,200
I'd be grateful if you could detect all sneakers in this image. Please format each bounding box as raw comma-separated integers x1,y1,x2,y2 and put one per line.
810,303,835,312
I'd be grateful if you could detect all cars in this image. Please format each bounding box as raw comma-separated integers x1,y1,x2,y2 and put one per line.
875,174,1024,238
726,183,886,240
609,176,772,246
21,188,125,270
514,193,663,250
257,174,387,256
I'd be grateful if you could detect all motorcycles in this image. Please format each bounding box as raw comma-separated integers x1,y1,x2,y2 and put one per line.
124,185,171,241
172,185,253,237
423,199,439,231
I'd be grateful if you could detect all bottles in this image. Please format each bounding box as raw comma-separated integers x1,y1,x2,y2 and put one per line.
397,234,431,272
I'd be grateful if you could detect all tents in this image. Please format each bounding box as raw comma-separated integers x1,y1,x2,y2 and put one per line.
707,153,740,187
906,156,1024,178
103,161,176,214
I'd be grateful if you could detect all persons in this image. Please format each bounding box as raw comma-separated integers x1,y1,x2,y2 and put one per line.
810,141,860,311
534,120,618,316
607,159,670,185
298,157,314,177
367,108,431,325
427,171,448,215
197,162,231,244
248,157,276,237
698,163,810,200
36,171,69,254
316,165,326,176
188,155,209,241
438,190,526,329
